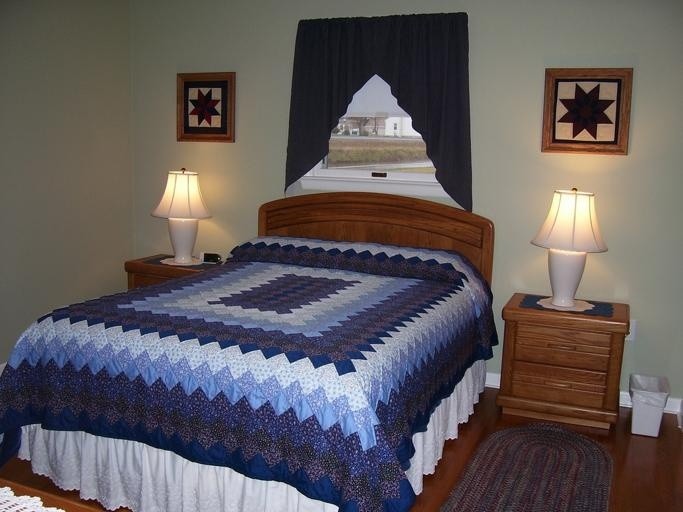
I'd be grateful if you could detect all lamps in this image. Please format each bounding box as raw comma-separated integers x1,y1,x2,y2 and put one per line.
530,187,610,308
151,168,212,264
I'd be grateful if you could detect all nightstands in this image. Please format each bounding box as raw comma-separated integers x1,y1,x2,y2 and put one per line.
124,255,217,290
496,293,630,430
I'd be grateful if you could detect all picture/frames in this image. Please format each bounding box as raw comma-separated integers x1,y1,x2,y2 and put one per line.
176,73,235,143
542,68,634,155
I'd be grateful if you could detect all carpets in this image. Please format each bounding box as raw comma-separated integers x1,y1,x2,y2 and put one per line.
439,422,614,512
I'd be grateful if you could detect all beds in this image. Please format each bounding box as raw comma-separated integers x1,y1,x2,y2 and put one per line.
10,193,494,511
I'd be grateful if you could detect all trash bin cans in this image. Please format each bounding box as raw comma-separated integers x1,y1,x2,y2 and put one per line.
629,374,670,438
